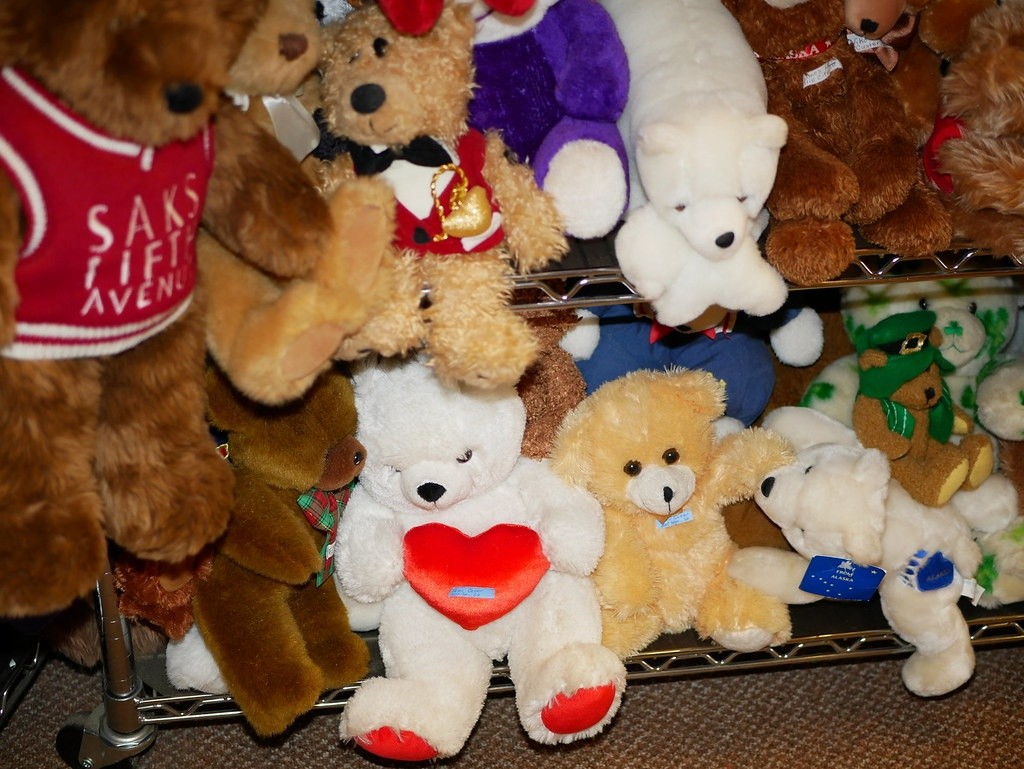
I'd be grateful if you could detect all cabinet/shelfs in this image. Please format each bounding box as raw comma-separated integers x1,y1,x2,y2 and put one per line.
55,228,1024,769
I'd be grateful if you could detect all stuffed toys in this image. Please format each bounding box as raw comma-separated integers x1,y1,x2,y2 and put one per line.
1,1,1023,765
